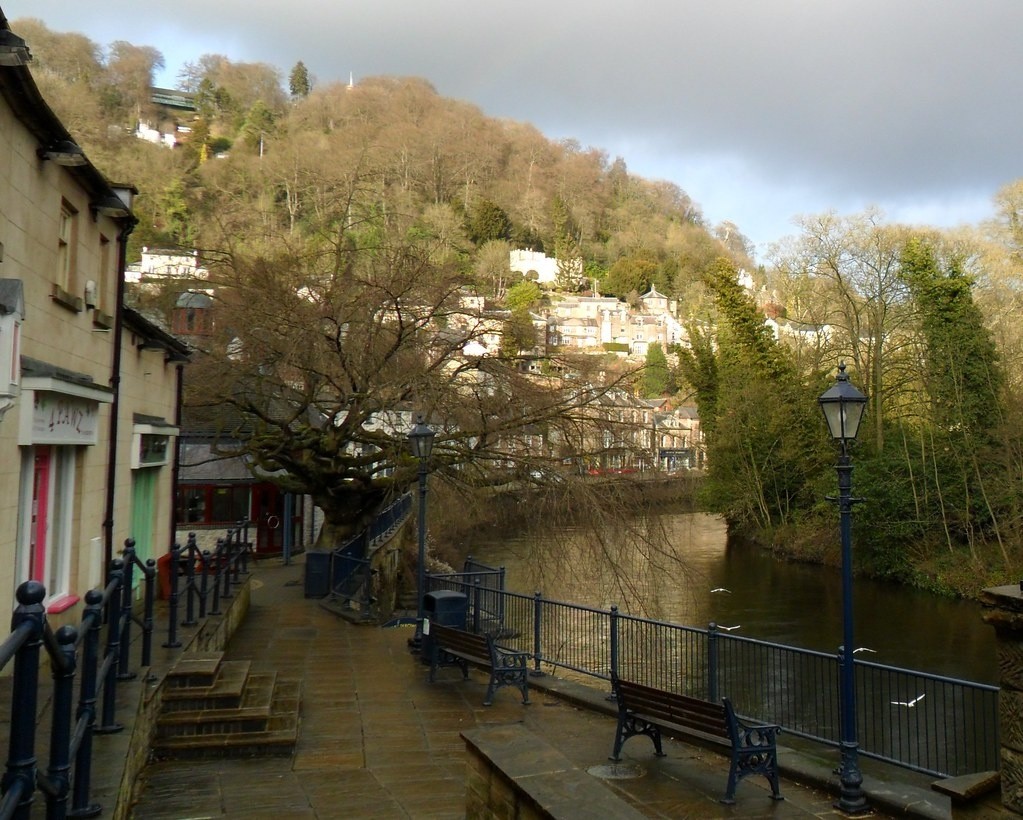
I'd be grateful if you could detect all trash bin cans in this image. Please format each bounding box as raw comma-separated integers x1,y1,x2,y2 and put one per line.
421,591,468,664
305,550,333,597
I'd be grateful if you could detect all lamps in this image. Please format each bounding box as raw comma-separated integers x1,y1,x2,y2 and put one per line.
88,195,130,218
35,139,89,169
137,340,167,354
165,355,191,367
0,28,33,66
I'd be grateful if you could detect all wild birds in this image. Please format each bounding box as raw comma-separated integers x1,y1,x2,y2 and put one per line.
717,624,740,631
853,648,877,654
891,694,925,707
711,588,732,594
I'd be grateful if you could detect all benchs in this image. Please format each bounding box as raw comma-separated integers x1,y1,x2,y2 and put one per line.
423,615,535,707
607,668,785,805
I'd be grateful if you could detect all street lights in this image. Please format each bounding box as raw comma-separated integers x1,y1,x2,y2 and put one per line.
407,414,436,644
817,360,871,815
